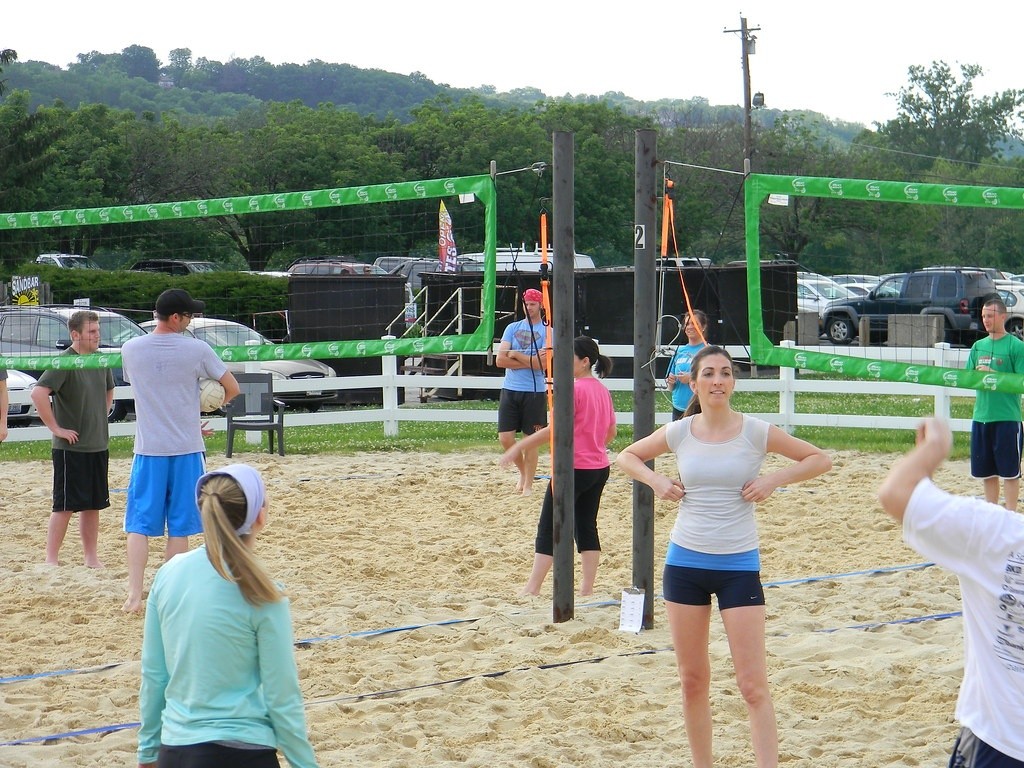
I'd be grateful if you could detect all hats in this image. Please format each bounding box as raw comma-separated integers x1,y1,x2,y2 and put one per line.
156,289,205,314
195,464,265,536
523,289,543,302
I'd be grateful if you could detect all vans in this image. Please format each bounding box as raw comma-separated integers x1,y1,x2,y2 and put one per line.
459,248,595,270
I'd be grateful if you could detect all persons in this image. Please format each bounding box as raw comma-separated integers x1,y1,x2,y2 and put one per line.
667,309,712,422
616,345,833,768
497,335,617,597
137,463,319,768
0,354,9,444
496,289,554,498
30,312,116,570
878,416,1024,768
121,289,241,611
964,299,1024,512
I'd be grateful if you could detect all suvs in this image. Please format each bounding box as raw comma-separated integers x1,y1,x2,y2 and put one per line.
289,258,387,274
656,257,716,267
823,268,997,345
374,257,430,271
388,260,483,287
0,303,153,422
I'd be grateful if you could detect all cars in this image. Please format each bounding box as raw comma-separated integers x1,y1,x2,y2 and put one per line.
113,318,338,412
131,260,222,275
986,268,1024,341
4,368,54,424
37,254,101,270
797,271,907,336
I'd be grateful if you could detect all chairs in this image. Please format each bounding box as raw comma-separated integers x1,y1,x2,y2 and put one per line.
225,372,286,459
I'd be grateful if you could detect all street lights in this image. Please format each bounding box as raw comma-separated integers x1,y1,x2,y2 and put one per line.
744,92,764,169
